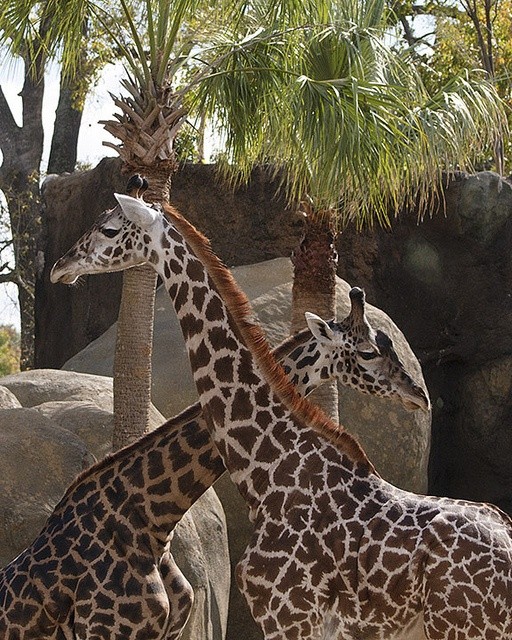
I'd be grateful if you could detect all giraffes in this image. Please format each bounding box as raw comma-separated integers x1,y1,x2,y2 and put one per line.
2,287,430,640
50,173,512,640
